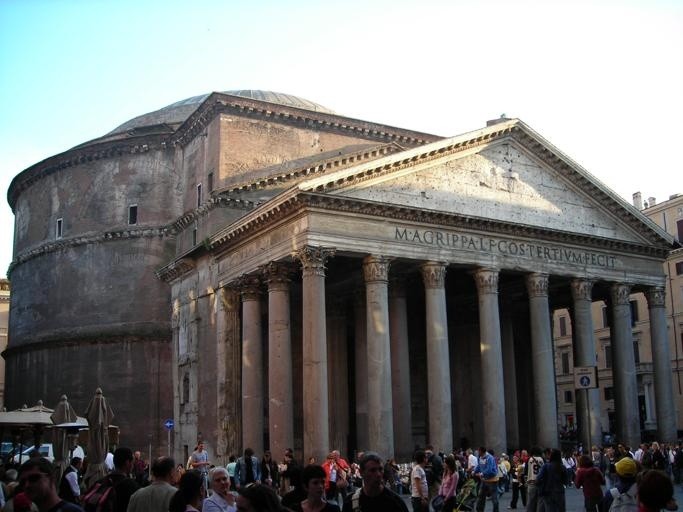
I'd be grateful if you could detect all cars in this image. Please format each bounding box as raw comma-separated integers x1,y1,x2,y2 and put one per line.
0,442,114,471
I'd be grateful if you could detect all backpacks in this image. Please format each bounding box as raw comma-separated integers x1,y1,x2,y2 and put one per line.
609,483,638,512
84,478,128,512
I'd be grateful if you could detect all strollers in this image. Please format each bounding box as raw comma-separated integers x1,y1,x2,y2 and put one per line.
453,473,479,512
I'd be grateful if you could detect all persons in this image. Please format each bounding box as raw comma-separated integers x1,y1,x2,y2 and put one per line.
1,437,682,512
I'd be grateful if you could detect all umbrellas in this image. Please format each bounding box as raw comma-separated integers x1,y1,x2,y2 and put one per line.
50,394,78,479
0,399,55,460
86,387,114,480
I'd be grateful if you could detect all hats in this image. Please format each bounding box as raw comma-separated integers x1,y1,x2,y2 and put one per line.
615,457,638,477
